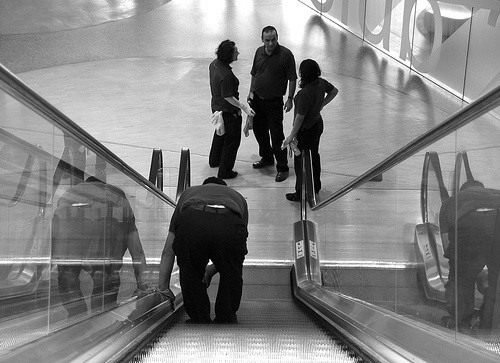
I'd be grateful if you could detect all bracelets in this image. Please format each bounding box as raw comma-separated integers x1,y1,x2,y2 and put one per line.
137,283,149,292
157,288,169,293
288,96,293,99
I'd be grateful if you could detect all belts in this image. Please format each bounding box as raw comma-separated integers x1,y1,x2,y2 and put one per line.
189,203,231,214
469,210,497,217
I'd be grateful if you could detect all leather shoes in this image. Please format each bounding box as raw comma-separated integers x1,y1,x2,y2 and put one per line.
253,160,274,168
275,171,289,181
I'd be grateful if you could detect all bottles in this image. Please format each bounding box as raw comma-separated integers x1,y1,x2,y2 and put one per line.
248,115,253,129
289,141,301,156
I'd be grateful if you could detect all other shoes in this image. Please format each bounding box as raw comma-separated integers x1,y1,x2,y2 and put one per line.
210,163,220,168
285,191,307,202
217,171,238,180
185,317,212,325
212,317,238,325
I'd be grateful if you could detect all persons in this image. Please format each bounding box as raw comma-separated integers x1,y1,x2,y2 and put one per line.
283,58,339,202
439,178,500,331
247,25,298,181
207,38,256,179
156,176,249,324
48,174,148,318
